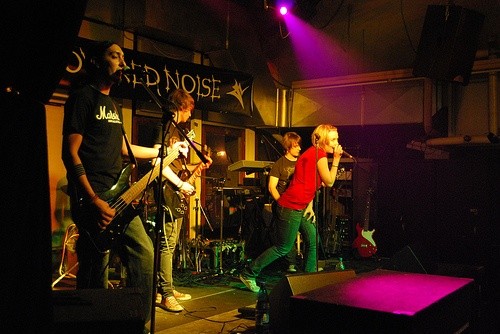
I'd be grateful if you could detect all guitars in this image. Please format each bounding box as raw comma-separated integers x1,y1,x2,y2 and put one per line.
84,129,195,252
162,145,212,219
352,185,377,257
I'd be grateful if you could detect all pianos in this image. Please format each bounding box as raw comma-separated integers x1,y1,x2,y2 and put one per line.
224,159,275,242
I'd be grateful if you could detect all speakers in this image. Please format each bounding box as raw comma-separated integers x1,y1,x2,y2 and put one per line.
267,268,357,334
383,241,431,274
0,286,150,334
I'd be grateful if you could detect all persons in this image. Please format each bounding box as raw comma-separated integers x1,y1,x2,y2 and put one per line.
240,124,344,292
268,132,302,273
62,41,189,334
152,89,213,312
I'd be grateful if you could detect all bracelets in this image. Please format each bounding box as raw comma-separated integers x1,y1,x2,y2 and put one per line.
68,163,86,178
88,195,99,205
331,165,339,167
176,180,182,186
178,181,184,189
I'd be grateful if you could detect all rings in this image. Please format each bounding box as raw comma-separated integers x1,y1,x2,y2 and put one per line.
340,153,342,155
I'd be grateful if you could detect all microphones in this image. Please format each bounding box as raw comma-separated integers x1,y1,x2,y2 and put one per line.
342,150,354,158
122,66,146,75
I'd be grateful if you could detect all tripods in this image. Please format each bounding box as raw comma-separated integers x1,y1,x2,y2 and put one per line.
193,180,241,283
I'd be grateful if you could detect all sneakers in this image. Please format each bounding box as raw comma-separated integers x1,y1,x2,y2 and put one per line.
157,290,191,300
160,297,183,311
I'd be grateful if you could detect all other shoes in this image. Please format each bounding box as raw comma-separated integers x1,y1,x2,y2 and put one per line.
239,274,261,292
